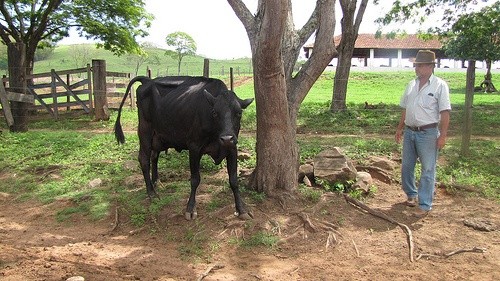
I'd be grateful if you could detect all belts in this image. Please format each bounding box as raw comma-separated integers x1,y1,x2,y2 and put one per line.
408,123,436,130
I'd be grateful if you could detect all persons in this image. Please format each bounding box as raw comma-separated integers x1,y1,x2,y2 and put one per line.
394,50,452,218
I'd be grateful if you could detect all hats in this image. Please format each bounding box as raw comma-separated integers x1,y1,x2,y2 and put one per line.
413,49,437,65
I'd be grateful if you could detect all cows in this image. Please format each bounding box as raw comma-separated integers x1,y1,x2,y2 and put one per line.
113,76,255,220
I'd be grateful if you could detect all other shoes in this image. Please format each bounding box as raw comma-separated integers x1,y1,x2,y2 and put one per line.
413,207,427,217
406,197,416,206
